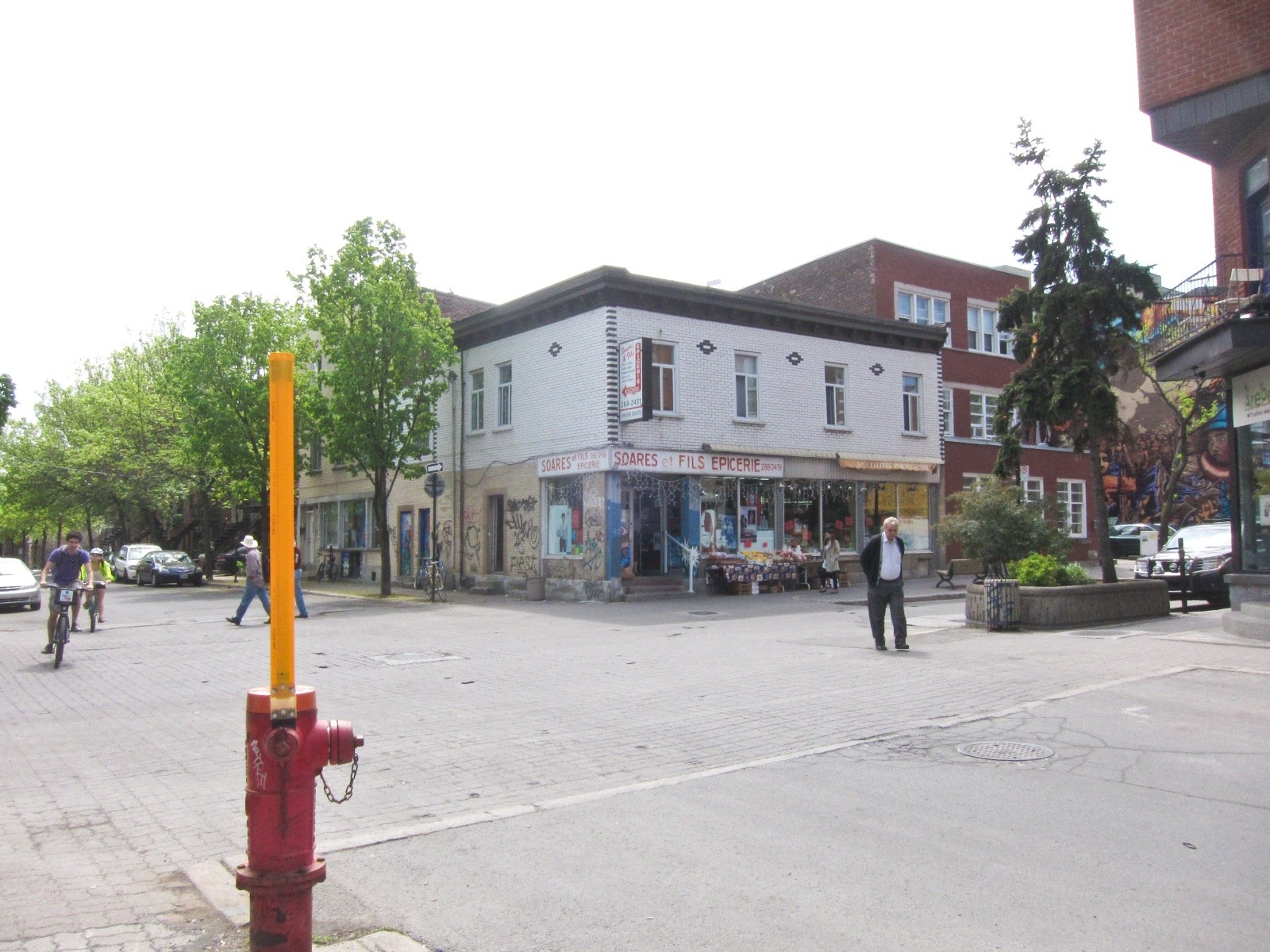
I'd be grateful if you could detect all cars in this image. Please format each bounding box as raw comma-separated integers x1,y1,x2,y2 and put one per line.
0,556,44,612
215,546,264,574
1104,523,1176,556
138,549,204,586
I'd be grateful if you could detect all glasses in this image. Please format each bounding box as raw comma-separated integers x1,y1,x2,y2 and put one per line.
886,527,897,533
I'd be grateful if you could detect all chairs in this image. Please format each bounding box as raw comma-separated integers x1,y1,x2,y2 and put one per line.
1214,269,1265,319
1175,301,1215,338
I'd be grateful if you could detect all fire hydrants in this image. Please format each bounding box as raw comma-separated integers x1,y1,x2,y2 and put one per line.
237,666,359,948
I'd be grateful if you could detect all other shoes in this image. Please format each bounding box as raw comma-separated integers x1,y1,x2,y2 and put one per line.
896,643,909,650
820,587,826,592
227,617,239,625
264,618,270,624
831,590,838,594
875,644,887,650
296,615,307,618
42,645,53,653
71,622,82,632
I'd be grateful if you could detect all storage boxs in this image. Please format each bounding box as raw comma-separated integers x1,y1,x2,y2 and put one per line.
729,580,781,595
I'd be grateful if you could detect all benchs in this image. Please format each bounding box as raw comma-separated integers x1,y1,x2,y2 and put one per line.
934,558,990,589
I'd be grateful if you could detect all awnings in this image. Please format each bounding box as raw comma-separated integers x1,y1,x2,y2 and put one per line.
703,442,945,475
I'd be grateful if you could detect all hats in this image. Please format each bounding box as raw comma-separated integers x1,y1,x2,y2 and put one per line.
240,535,259,549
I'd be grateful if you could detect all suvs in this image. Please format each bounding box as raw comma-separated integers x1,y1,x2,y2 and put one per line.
115,543,161,584
1132,518,1246,603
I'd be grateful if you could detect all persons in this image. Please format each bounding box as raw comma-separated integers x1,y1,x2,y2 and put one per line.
723,509,756,549
858,516,910,651
41,530,94,654
819,530,842,594
78,546,115,623
556,513,569,554
225,534,271,627
294,539,309,618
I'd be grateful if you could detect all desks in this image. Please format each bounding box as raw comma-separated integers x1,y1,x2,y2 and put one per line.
1180,285,1235,330
703,556,838,591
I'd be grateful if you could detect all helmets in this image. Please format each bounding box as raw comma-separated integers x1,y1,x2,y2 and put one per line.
90,548,104,555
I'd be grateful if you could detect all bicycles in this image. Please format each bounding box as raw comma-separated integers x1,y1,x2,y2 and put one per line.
39,582,94,668
422,561,447,602
315,553,339,583
412,554,432,591
81,580,110,630
